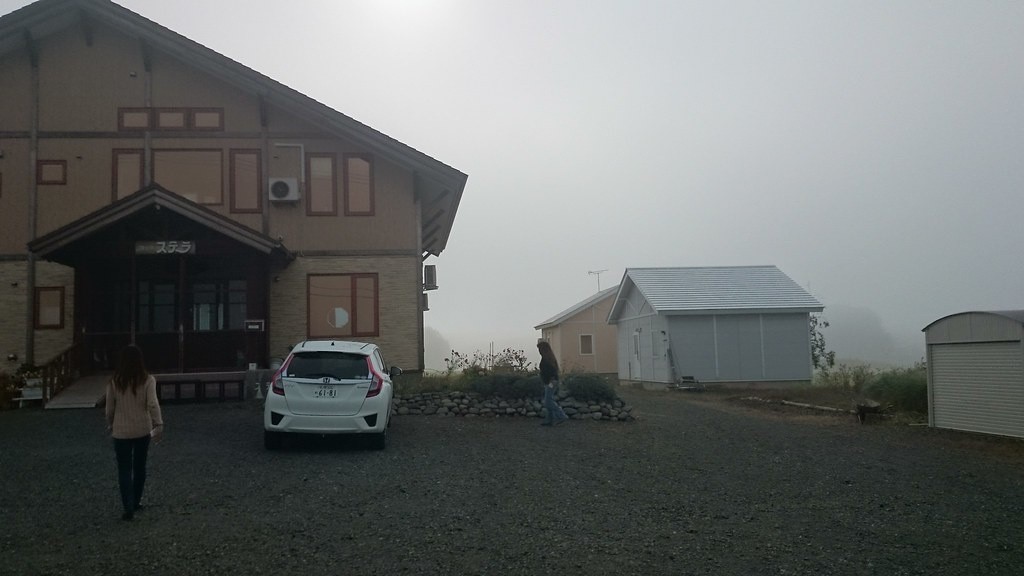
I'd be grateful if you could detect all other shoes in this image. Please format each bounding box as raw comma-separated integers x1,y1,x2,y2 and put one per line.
134,503,142,510
555,420,564,425
541,423,551,426
123,513,134,521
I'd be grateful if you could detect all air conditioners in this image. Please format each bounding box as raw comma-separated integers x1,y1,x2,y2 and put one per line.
422,265,438,290
268,176,300,205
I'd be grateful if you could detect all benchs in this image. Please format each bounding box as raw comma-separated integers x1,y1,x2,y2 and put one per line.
150,371,251,404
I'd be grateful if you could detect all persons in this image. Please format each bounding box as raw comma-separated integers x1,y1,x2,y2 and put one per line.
104,344,163,522
537,341,570,427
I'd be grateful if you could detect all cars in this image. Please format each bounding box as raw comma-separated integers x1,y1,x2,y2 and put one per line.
264,337,404,450
